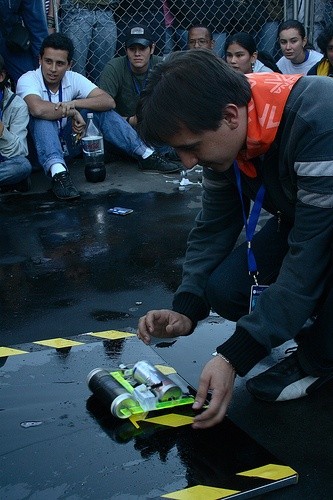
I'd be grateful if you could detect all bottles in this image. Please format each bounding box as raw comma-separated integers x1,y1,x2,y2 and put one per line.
80,113,106,183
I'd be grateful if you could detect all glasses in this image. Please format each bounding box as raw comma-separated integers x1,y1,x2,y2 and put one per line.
188,39,212,45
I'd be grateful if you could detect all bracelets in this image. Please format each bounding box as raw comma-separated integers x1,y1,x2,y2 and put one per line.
211,350,238,379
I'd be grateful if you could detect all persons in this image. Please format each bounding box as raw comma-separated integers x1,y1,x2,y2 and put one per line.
0,0,118,90
137,49,333,430
275,19,330,76
306,24,333,77
16,32,182,200
224,33,284,74
188,24,216,52
0,55,33,186
98,27,182,163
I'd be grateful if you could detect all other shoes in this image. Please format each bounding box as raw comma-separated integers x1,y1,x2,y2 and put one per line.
10,178,32,192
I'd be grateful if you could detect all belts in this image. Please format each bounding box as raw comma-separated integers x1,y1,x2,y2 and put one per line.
73,3,111,11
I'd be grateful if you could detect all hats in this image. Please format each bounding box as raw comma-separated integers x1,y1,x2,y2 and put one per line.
126,24,153,47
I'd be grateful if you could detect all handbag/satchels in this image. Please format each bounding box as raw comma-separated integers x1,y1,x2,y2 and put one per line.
7,23,34,56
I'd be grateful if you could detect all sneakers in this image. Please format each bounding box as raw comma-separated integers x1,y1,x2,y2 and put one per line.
141,151,181,173
51,170,80,199
246,346,333,402
